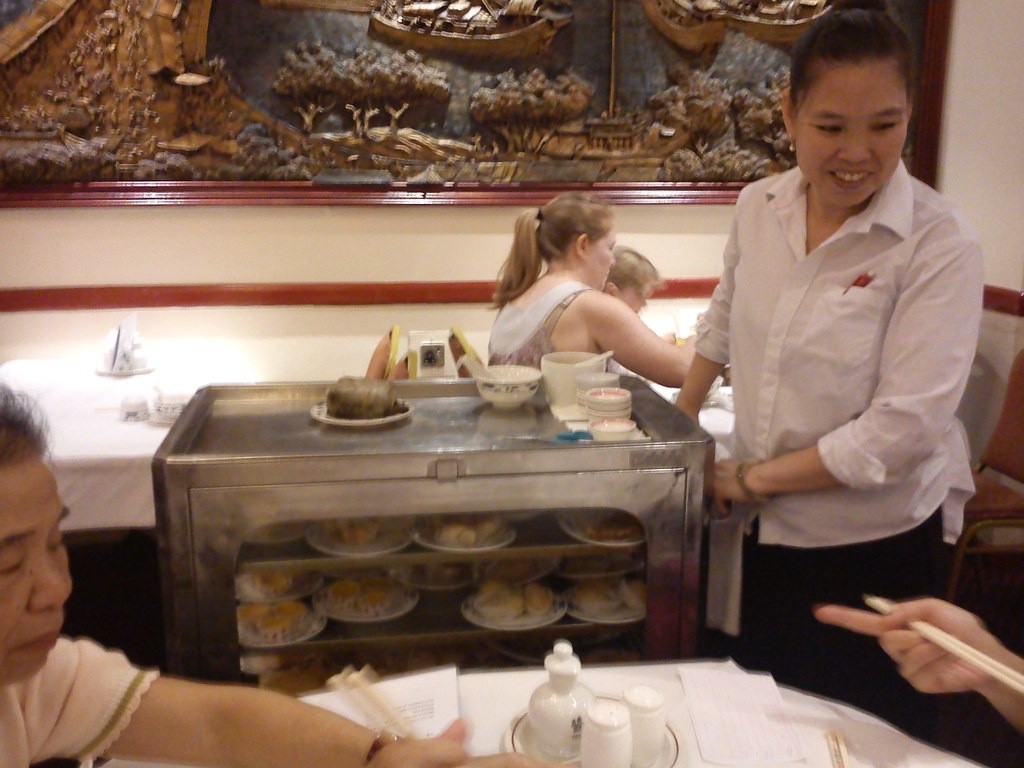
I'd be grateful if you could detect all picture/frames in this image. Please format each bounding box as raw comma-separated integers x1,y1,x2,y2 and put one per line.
0,0,955,207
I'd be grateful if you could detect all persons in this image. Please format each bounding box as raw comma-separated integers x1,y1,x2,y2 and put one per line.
487,191,698,390
677,8,986,724
0,384,542,768
816,599,1024,733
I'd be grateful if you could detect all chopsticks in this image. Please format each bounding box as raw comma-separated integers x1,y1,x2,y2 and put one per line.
826,730,848,768
865,593,1024,695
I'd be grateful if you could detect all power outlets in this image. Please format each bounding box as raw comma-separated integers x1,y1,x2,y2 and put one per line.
421,344,444,369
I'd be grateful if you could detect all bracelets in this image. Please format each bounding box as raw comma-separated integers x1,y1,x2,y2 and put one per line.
736,458,767,505
367,732,402,761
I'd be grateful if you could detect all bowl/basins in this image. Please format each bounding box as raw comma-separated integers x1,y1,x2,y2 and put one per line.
473,364,543,407
540,350,636,442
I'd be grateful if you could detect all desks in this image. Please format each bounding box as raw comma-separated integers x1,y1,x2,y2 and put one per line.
1,333,486,537
96,656,996,768
608,327,751,639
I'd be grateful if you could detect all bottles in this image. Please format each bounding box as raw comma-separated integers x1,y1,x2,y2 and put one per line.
529,641,587,758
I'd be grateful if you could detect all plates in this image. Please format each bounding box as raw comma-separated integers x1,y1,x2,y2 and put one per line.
236,507,651,690
505,695,680,768
311,400,412,426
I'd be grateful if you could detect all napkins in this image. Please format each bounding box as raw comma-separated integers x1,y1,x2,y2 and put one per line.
677,667,805,764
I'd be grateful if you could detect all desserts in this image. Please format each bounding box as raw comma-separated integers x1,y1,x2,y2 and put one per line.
235,504,645,641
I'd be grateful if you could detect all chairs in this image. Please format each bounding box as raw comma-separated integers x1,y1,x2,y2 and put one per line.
945,347,1024,602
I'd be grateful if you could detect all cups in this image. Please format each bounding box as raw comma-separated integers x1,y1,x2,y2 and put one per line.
580,689,666,766
